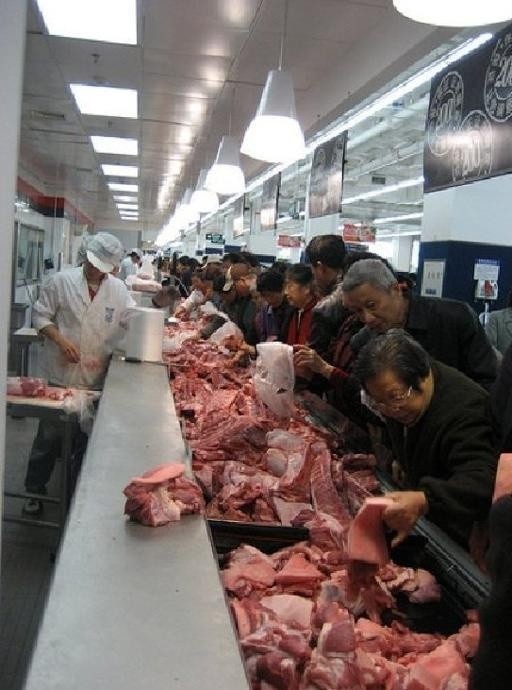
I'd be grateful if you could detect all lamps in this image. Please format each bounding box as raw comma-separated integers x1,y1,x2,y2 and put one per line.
153,0,307,248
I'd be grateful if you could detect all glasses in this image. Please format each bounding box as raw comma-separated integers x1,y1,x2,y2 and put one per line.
372,386,412,410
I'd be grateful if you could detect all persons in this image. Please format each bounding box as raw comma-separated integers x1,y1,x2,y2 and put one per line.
354,332,495,548
467,453,512,690
120,236,500,408
21,231,135,519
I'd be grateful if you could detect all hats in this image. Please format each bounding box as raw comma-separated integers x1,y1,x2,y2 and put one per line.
87,233,122,273
223,263,248,291
